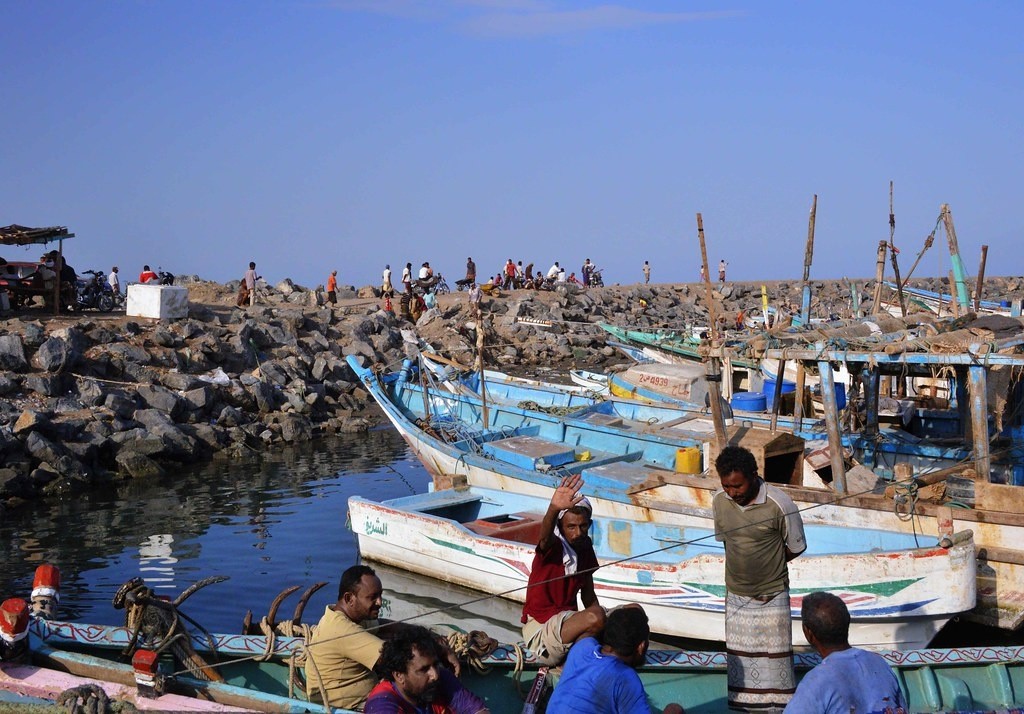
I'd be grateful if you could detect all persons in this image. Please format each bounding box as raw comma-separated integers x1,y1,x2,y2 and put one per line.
237,262,262,306
108,267,125,308
364,626,490,713
21,250,77,313
712,446,807,714
0,265,37,306
519,473,645,665
468,282,482,316
781,591,907,713
306,566,386,710
700,265,705,282
718,260,728,282
379,261,439,325
642,261,650,285
487,259,595,290
322,271,340,306
466,257,475,287
140,265,159,284
738,308,774,331
543,602,651,714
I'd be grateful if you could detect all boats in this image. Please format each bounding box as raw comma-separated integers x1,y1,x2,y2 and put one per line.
1,181,1024,714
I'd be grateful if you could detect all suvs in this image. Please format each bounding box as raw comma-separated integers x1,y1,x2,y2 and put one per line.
0,262,77,311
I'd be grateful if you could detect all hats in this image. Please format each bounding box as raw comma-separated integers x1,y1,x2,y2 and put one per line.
558,493,592,520
113,266,120,271
718,314,727,322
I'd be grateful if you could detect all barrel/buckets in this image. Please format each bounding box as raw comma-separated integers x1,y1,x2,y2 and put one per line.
834,382,846,410
676,447,702,473
730,392,767,413
762,378,796,411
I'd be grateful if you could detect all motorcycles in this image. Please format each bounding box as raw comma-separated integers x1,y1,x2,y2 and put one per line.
588,268,604,289
410,274,448,296
76,269,114,313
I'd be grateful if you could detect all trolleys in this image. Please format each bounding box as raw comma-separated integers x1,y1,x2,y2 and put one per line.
455,275,475,292
